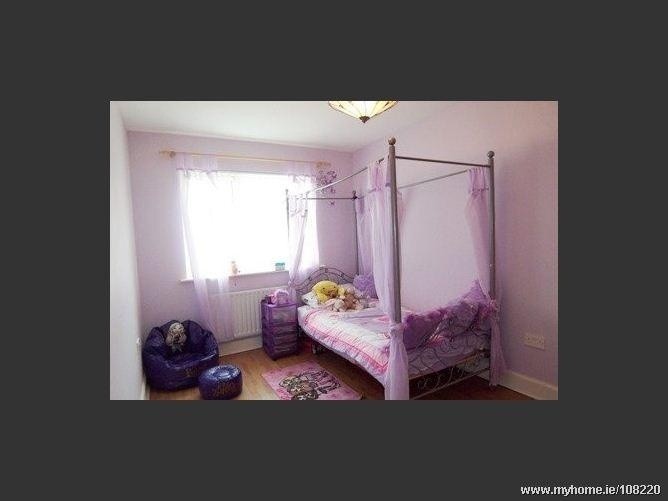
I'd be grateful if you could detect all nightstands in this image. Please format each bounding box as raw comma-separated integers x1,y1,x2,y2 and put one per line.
260,300,299,361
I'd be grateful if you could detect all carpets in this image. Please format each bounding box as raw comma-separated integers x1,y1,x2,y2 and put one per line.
261,361,362,400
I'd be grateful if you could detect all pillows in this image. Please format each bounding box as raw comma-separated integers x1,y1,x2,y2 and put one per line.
300,283,363,310
382,278,494,353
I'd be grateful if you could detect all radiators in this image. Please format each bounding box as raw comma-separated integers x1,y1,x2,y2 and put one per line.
207,285,288,345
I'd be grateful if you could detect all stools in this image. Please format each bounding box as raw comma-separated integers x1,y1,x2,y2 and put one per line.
199,365,242,400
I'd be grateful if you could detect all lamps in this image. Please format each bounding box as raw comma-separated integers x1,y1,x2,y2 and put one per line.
328,101,398,124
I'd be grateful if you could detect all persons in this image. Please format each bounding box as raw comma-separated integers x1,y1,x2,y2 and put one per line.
166,322,187,352
278,371,341,400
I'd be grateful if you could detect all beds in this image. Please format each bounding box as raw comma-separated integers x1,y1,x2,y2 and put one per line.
290,266,497,401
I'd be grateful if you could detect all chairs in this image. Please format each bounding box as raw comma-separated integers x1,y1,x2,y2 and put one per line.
142,320,219,391
